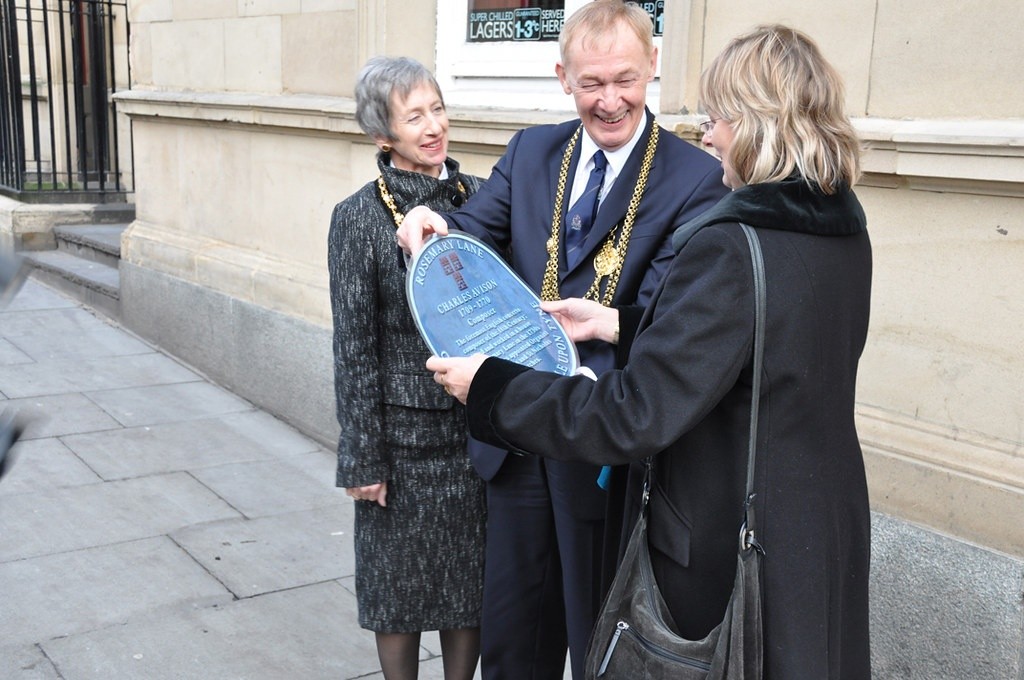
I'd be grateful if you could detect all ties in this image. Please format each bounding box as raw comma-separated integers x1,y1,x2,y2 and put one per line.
564,150,610,271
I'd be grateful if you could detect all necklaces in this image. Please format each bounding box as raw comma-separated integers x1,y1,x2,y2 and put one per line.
539,117,659,309
376,172,465,226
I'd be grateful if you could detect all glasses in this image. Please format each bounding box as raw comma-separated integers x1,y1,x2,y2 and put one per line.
699,117,723,136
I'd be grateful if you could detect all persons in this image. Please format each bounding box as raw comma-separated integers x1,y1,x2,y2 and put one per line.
388,0,732,680
326,60,489,680
424,22,875,680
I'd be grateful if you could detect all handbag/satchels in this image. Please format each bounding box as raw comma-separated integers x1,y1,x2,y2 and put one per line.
583,460,765,680
